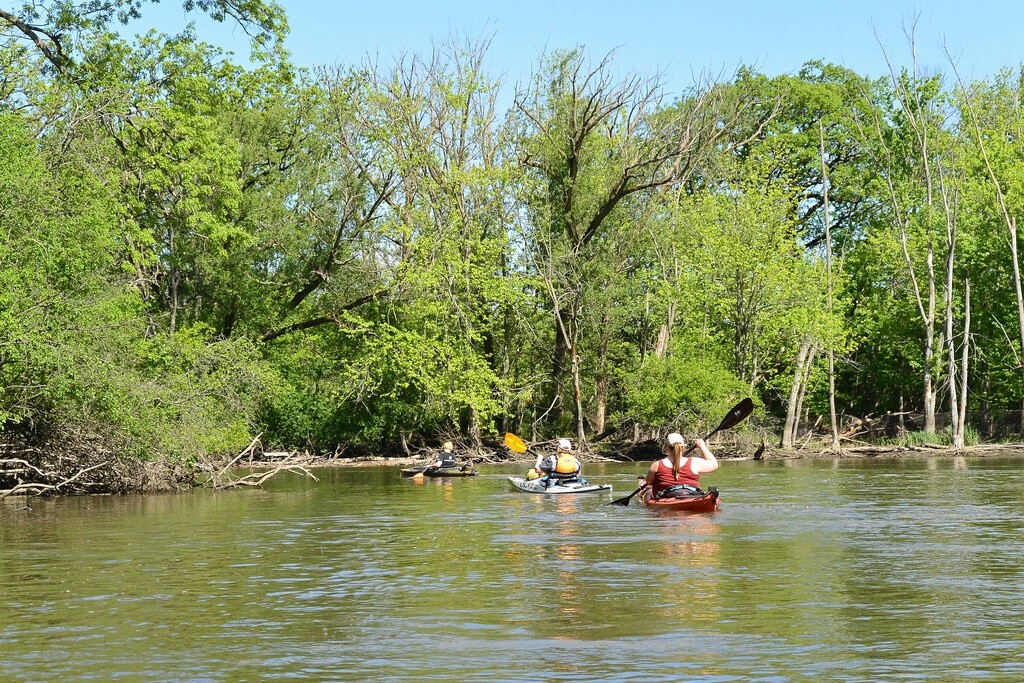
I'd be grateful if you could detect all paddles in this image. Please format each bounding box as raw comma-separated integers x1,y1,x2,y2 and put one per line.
413,459,434,478
504,431,546,460
603,398,754,506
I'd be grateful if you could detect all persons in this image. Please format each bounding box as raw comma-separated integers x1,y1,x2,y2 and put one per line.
535,439,581,487
425,442,456,468
646,433,718,500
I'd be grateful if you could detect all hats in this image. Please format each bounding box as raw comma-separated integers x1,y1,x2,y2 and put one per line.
556,439,572,451
440,442,453,450
667,433,684,447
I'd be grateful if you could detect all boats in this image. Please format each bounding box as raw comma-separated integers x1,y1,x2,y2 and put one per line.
399,456,480,476
507,461,614,493
636,473,721,513
